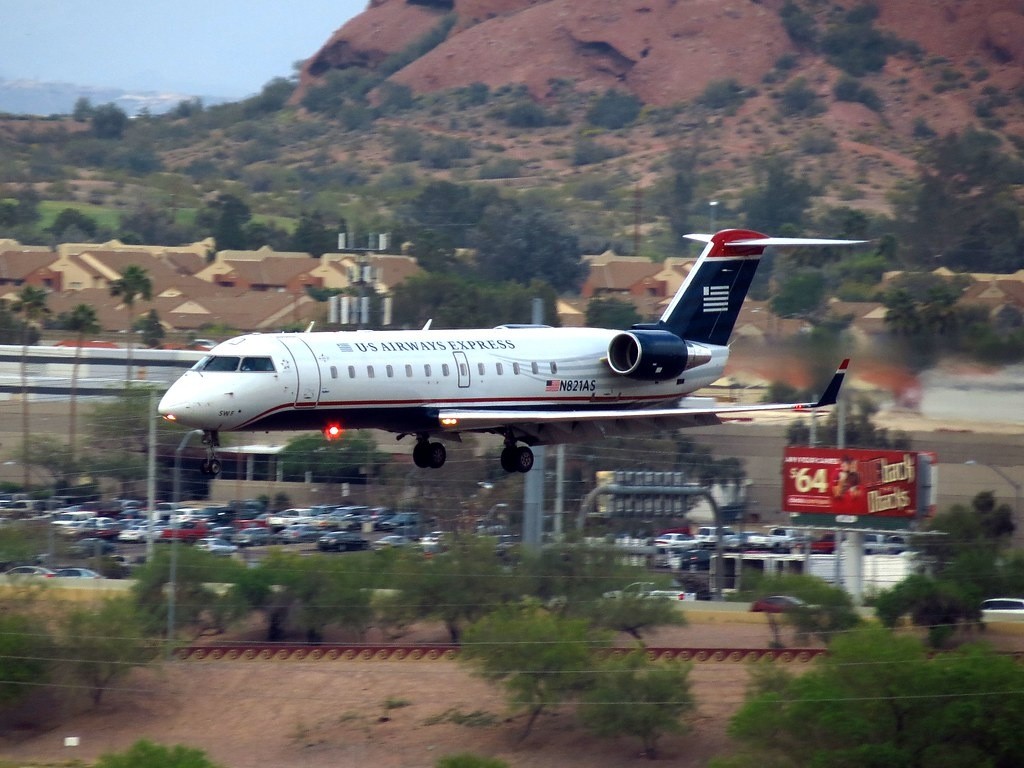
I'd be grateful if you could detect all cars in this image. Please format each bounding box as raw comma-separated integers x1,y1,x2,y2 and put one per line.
0,484,918,580
752,594,821,616
976,597,1024,624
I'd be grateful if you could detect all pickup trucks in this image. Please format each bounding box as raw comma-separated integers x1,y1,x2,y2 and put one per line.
603,582,702,600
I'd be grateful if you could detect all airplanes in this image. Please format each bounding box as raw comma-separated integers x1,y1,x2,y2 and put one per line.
157,227,875,474
